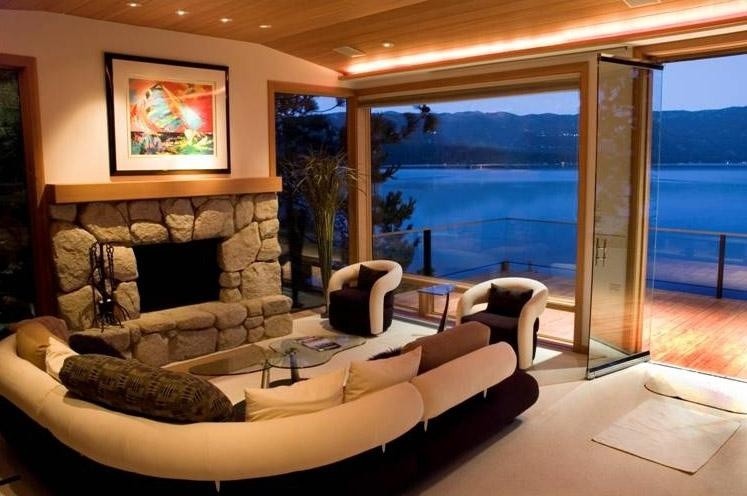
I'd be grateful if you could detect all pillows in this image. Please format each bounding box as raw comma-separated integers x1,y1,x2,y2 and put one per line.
358,264,388,289
15,320,63,372
59,354,237,421
346,347,423,399
243,368,345,422
401,321,490,376
486,283,533,317
45,336,79,382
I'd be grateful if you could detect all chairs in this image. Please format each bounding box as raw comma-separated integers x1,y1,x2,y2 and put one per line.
456,277,549,370
325,260,402,338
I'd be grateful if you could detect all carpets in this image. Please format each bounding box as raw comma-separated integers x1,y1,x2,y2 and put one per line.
644,376,746,416
590,398,740,475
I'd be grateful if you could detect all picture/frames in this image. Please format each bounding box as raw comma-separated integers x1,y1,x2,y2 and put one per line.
103,52,231,177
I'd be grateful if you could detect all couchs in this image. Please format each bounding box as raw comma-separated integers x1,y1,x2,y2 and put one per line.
2,316,539,496
